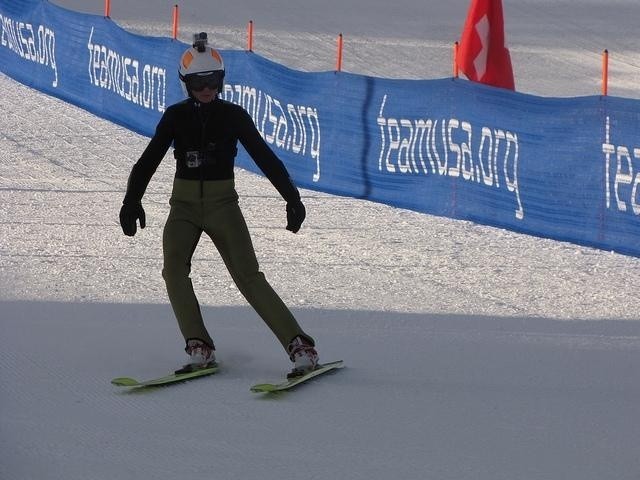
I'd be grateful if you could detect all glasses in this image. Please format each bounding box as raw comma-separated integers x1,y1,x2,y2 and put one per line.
178,68,225,91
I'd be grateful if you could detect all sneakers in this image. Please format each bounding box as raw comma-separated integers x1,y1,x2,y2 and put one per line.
290,336,319,372
183,339,216,371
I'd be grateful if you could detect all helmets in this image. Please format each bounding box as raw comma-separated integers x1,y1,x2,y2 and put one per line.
179,46,225,97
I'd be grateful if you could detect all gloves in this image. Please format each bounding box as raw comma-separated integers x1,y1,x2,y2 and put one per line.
285,192,306,233
120,196,146,236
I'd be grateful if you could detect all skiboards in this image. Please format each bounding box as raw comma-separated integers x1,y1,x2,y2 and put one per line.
110,361,345,393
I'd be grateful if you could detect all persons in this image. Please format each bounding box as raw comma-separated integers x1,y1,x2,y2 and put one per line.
120,46,320,378
